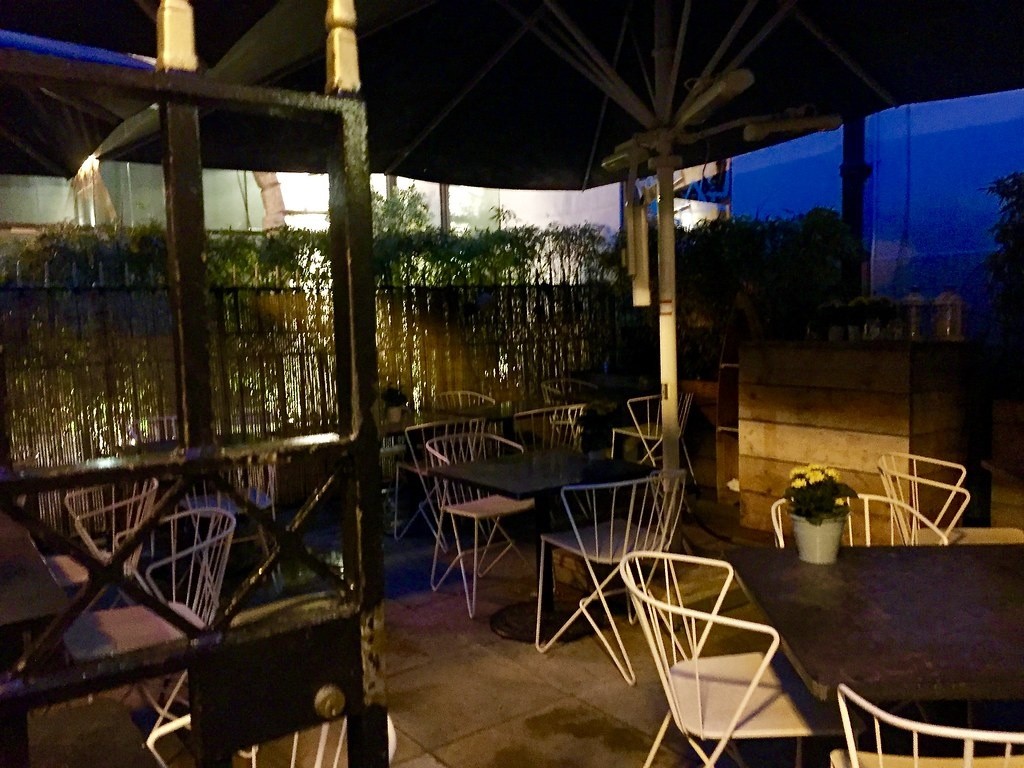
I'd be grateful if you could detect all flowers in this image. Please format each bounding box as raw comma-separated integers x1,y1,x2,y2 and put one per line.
784,462,860,523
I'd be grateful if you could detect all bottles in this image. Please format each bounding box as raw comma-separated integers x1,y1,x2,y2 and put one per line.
932,285,968,343
901,284,931,342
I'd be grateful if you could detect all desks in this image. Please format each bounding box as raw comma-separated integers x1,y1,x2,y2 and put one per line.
722,540,1023,756
428,452,664,640
0,513,72,768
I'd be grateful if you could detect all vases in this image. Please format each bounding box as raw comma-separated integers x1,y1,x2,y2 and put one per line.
792,515,844,565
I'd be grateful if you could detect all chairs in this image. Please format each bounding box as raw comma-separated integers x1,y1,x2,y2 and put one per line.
0,393,1024,767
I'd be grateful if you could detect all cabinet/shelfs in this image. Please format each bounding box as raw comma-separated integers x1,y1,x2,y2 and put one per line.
739,342,965,533
717,293,756,508
